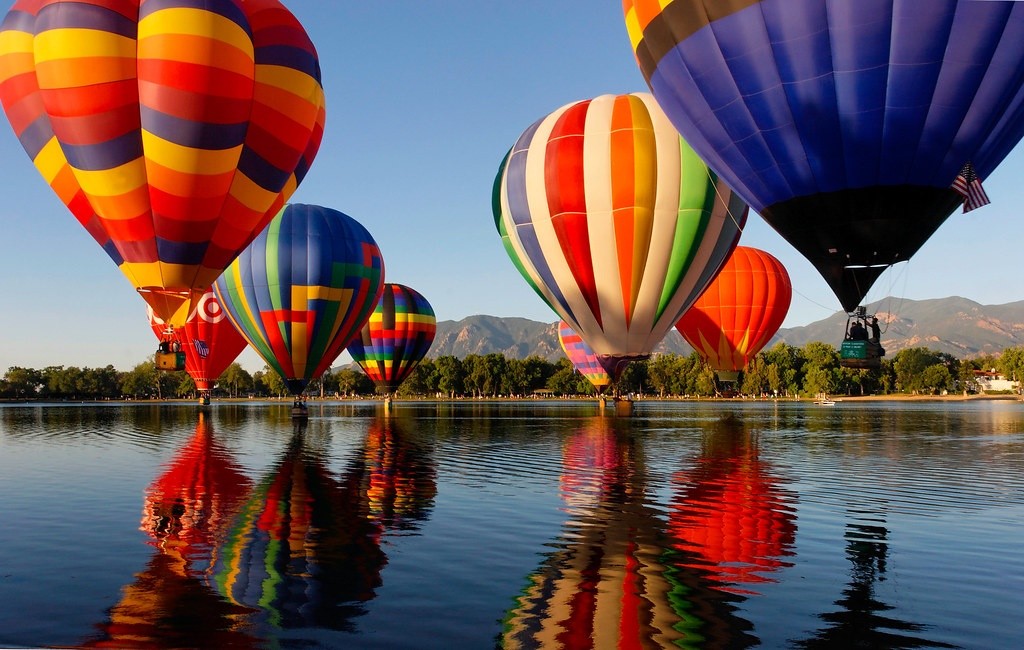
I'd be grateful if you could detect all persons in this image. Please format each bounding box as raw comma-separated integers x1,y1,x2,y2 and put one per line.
847,317,881,343
159,339,180,353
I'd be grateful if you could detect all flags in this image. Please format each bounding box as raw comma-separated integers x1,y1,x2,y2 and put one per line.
194,339,209,358
951,162,991,214
